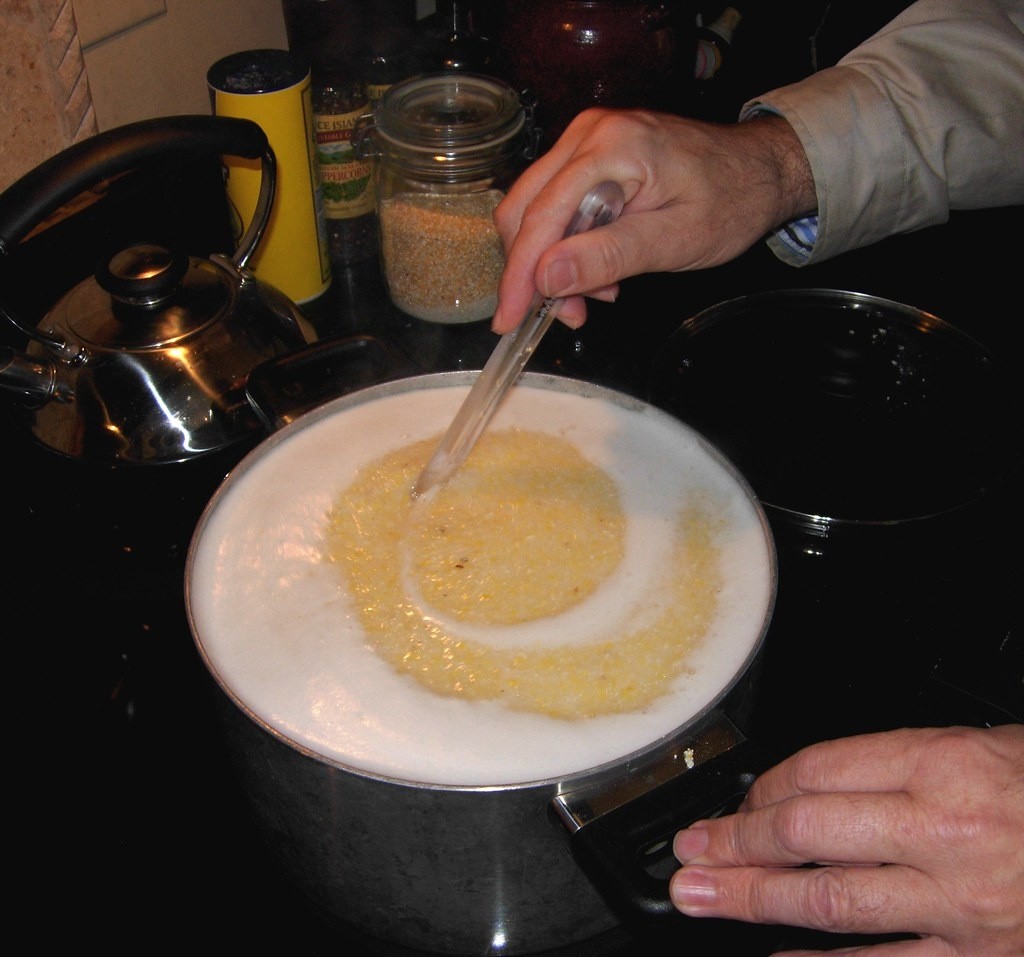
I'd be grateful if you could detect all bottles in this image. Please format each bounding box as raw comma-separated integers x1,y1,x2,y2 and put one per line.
414,0,506,91
367,52,407,250
349,72,543,324
310,56,383,268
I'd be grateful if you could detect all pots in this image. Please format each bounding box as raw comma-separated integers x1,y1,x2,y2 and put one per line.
184,335,781,938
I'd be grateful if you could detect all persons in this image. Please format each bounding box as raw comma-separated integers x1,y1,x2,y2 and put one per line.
492,0,1024,957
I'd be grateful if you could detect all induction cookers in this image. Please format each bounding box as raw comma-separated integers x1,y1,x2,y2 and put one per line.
0,147,1024,957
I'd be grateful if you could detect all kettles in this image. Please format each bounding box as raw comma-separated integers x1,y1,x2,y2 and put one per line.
0,115,321,469
